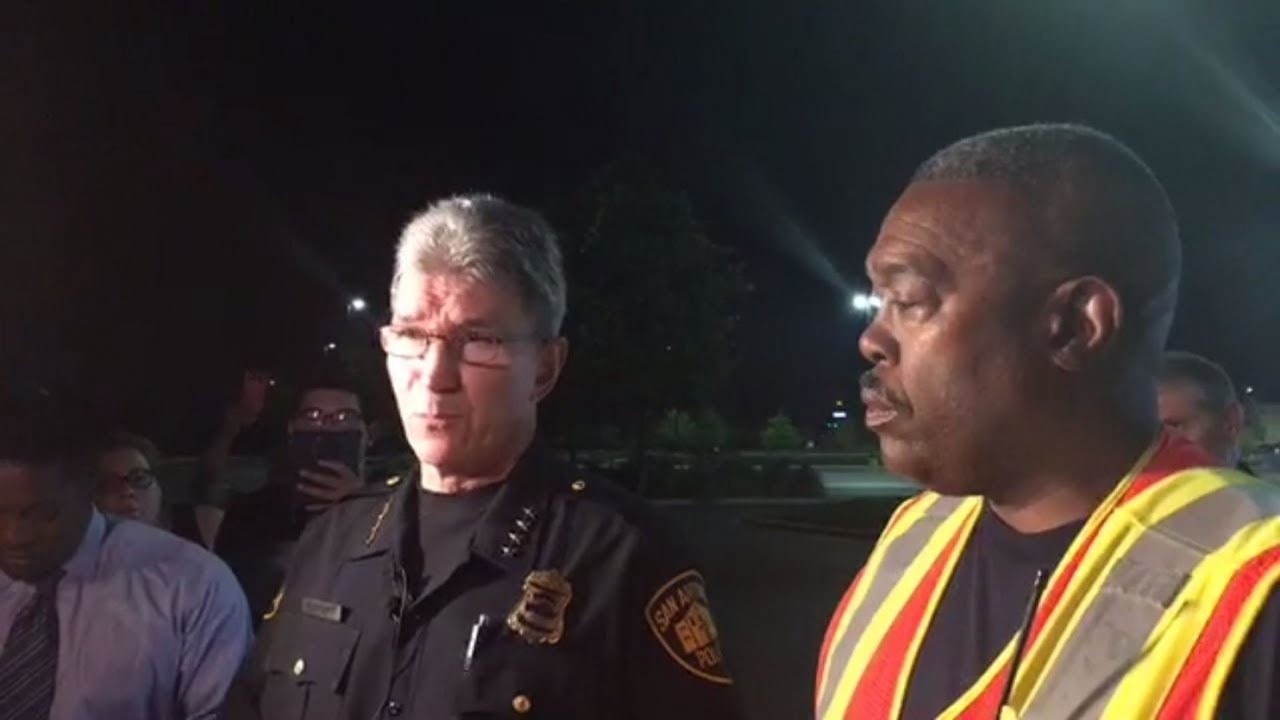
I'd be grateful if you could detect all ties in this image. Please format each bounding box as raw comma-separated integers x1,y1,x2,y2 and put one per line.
0,573,59,719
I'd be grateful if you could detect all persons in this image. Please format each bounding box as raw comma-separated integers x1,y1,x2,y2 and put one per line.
1152,352,1251,474
193,365,386,612
259,192,735,719
0,408,252,720
811,122,1279,719
78,430,179,532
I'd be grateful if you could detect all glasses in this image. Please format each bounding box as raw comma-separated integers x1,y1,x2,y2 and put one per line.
98,469,153,494
302,406,359,429
381,324,548,361
